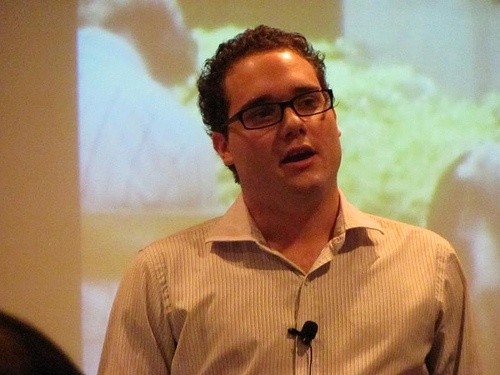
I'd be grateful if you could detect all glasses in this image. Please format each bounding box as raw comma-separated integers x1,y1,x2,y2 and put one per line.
223,88,335,129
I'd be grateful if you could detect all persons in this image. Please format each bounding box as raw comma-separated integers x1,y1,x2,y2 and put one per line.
96,24,483,375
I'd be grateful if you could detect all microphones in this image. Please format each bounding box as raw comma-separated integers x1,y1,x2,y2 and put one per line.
287,320,318,345
